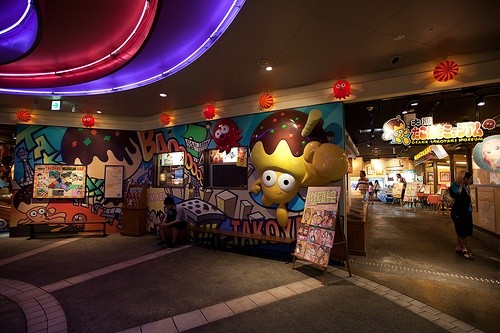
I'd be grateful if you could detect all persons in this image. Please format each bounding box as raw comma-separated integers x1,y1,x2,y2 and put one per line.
396,174,406,204
449,170,474,260
159,196,187,248
326,131,335,144
368,182,374,204
374,180,380,198
355,171,369,200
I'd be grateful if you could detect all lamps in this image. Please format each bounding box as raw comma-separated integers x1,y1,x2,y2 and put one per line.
71,104,80,113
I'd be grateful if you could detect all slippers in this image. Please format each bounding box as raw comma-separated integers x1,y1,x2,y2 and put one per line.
456,250,461,253
461,250,472,258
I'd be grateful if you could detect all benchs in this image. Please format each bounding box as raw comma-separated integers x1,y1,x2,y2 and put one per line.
187,226,296,264
25,221,110,239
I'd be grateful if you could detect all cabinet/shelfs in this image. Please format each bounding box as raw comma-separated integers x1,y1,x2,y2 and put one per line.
120,206,146,237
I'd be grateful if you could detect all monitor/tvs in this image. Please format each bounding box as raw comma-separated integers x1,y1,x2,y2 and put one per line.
210,164,248,188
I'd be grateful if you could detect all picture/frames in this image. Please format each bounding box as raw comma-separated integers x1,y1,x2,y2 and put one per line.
104,165,124,199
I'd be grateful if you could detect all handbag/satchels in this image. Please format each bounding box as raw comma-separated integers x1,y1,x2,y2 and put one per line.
442,186,462,206
